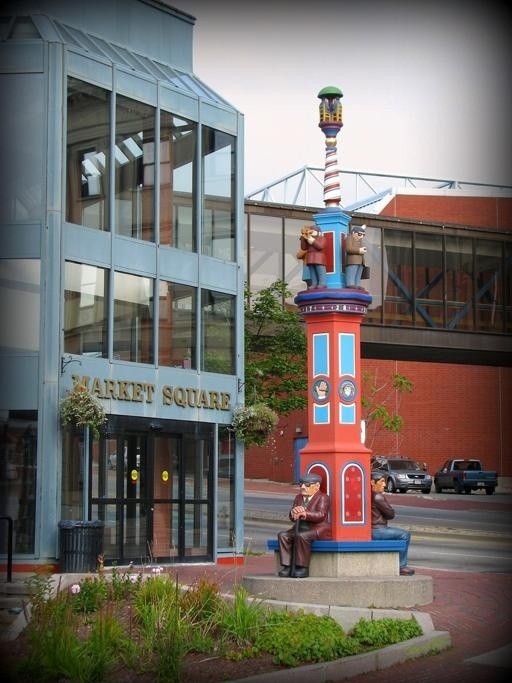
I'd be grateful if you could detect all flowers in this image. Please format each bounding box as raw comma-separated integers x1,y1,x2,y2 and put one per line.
60,380,108,441
227,401,280,450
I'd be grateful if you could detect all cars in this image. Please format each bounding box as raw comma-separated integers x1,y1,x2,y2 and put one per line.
371,456,432,494
108,447,141,470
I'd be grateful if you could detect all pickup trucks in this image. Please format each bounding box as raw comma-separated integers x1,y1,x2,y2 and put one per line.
434,458,498,495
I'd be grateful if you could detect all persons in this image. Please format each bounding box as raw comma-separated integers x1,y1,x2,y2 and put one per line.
277,473,330,577
344,226,367,291
296,225,313,290
300,225,328,289
370,471,415,576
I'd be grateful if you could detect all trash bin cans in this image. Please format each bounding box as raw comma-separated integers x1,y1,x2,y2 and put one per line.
59,520,105,573
294,436,309,484
